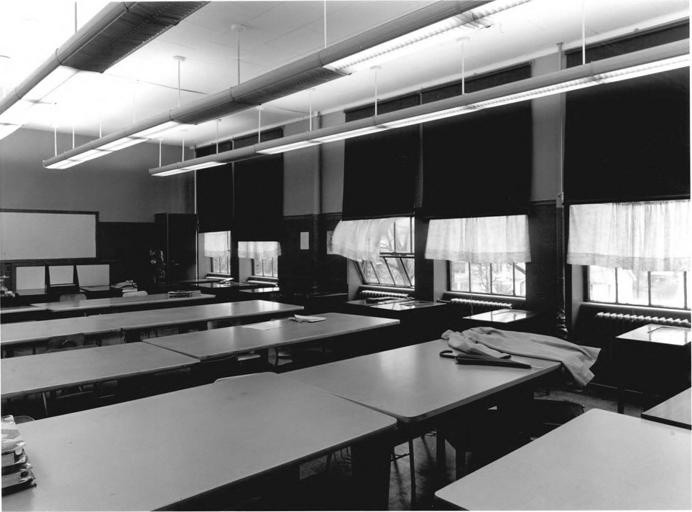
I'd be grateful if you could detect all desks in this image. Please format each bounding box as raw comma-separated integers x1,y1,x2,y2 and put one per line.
2,284,691,512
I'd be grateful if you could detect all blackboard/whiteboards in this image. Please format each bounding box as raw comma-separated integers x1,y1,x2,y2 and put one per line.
0,209,100,261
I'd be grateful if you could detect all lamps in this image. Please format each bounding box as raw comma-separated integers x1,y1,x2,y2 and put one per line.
1,0,690,176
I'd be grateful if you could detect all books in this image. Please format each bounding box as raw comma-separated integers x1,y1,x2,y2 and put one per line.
2,414,36,496
113,280,138,297
168,289,202,297
294,313,326,323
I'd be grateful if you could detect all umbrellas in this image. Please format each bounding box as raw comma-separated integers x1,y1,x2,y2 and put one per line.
439,349,532,369
